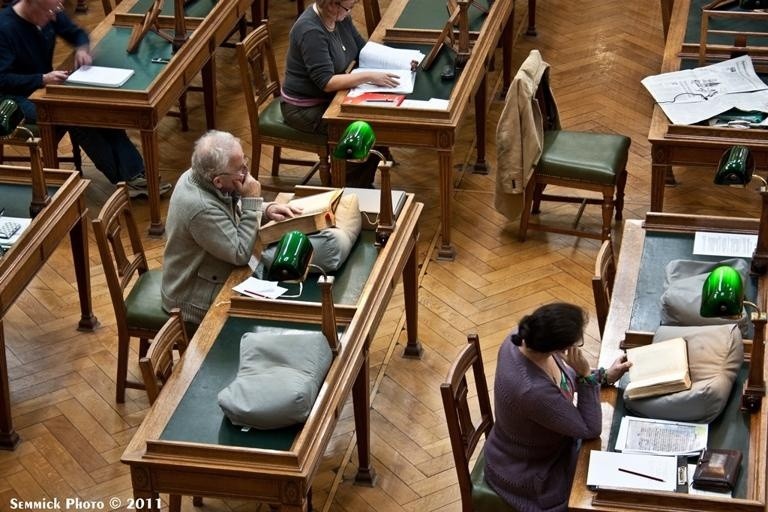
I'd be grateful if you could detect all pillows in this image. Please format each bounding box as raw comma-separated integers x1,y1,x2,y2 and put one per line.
622,258,749,425
216,327,334,432
263,189,362,272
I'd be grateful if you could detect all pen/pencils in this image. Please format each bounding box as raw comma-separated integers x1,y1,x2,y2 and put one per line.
244,290,271,299
366,99,394,102
618,468,664,482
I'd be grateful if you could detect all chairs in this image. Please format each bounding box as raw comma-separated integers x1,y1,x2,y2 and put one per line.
592,224,616,340
91,182,204,402
140,306,204,512
118,185,423,511
441,334,574,512
514,50,631,243
234,19,331,186
363,0,381,40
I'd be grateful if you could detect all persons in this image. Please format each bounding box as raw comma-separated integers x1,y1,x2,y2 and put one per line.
278,0,401,191
480,301,633,512
157,128,305,329
1,0,173,205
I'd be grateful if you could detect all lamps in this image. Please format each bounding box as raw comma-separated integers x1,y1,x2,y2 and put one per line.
0,100,52,219
267,229,342,356
332,120,397,249
702,265,768,415
713,145,768,277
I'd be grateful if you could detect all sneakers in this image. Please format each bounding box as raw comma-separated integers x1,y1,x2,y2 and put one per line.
119,174,173,198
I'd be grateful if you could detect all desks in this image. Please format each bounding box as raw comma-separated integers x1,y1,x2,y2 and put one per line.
646,0,768,213
0,165,100,452
319,0,538,262
567,220,768,511
24,0,269,235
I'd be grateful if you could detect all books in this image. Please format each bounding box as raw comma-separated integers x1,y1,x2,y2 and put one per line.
258,189,344,247
64,61,135,91
620,337,692,401
348,38,424,94
